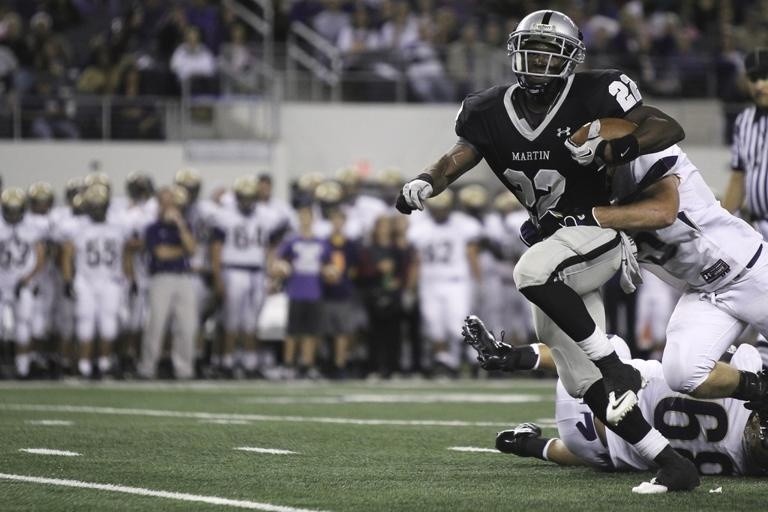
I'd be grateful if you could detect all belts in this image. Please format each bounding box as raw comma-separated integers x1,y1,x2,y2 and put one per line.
735,242,763,280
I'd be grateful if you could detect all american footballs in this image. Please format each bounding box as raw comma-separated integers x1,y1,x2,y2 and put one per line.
570,119,639,146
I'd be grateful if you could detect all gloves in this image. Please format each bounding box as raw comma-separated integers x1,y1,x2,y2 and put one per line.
395,172,434,215
518,219,544,247
586,140,617,194
545,206,599,229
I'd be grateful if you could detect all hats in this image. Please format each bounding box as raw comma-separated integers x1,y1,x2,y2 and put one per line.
743,47,768,77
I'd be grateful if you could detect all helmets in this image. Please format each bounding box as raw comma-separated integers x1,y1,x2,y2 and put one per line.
493,188,521,216
458,184,489,212
1,168,403,222
507,9,588,95
427,186,452,223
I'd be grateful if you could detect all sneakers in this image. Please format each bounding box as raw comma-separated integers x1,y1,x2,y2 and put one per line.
629,457,700,495
13,351,124,381
742,369,768,449
495,420,542,456
461,312,511,372
603,362,648,427
204,351,322,381
